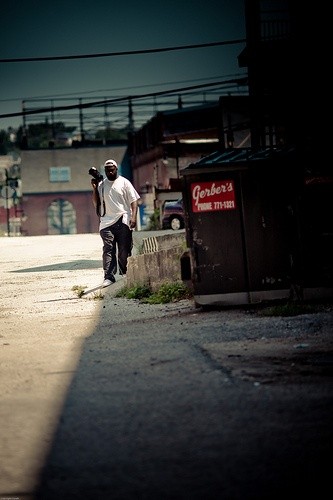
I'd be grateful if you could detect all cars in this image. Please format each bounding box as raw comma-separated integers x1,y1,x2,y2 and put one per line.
163,196,188,231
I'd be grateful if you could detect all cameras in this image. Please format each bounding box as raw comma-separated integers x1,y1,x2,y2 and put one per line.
89,166,103,182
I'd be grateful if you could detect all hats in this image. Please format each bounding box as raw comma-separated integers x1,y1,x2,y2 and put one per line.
104,159,118,169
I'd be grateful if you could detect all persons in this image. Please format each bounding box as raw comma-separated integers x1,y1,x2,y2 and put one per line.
90,158,140,288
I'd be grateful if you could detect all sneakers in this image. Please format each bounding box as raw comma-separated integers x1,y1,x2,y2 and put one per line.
102,279,113,288
119,274,126,279
